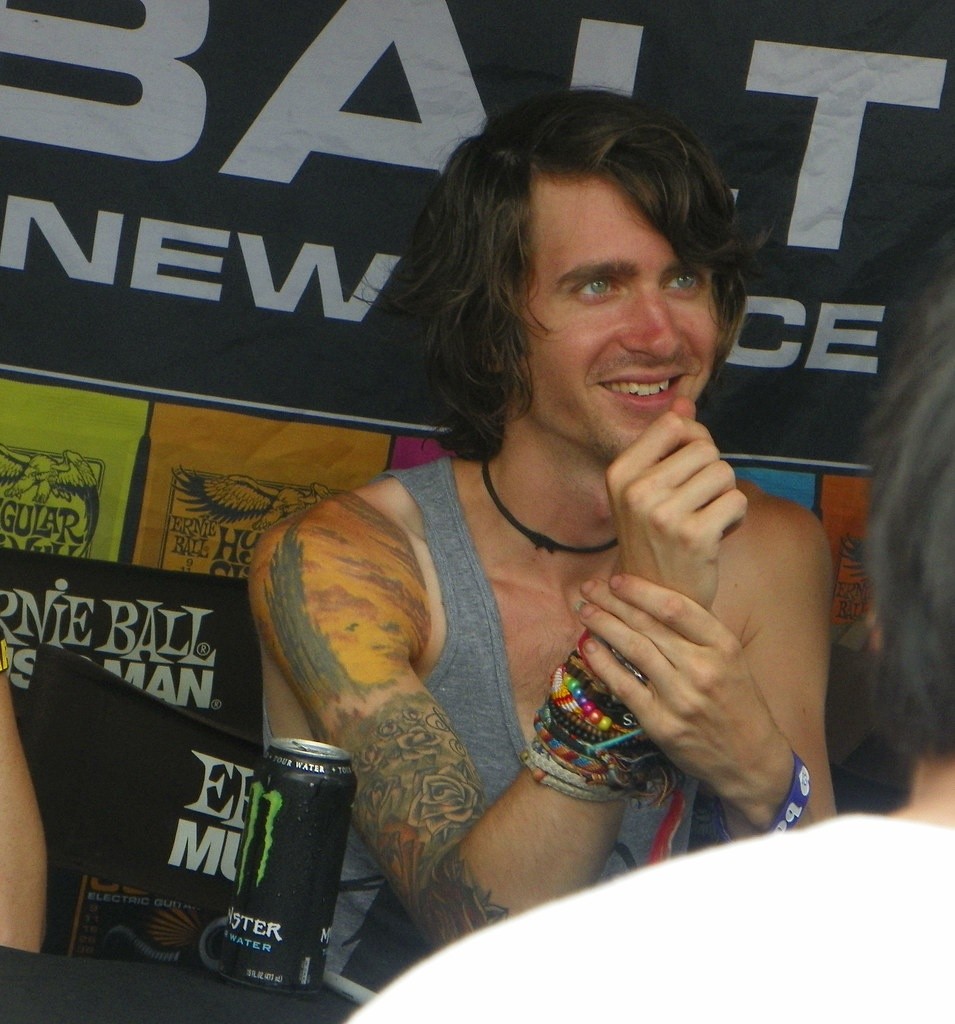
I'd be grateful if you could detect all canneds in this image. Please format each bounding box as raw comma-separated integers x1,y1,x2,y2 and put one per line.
215,736,357,1000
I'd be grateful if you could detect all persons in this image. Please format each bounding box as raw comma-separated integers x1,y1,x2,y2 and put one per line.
248,89,835,1002
343,223,954,1020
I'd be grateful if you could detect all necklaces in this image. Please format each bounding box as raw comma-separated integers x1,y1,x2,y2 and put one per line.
483,457,619,552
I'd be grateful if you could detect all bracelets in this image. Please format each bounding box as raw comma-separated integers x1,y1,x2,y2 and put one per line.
520,626,677,802
713,749,811,844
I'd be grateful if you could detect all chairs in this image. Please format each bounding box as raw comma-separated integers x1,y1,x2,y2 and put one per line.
27,638,261,975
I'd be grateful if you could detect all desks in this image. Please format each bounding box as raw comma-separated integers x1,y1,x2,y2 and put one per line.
1,944,362,1024
